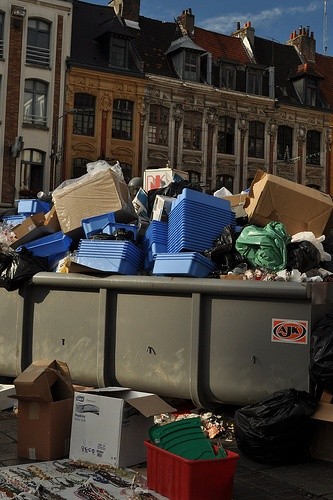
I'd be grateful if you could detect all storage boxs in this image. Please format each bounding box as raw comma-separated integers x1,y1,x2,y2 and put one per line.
144,439,240,500
7,395,74,462
2,167,250,277
13,358,75,401
243,170,333,239
309,391,333,461
69,385,178,469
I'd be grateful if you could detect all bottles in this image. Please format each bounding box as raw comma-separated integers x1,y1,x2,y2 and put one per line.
233,263,247,274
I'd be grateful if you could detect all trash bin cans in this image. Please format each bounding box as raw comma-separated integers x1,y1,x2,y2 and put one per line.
0,161,333,416
